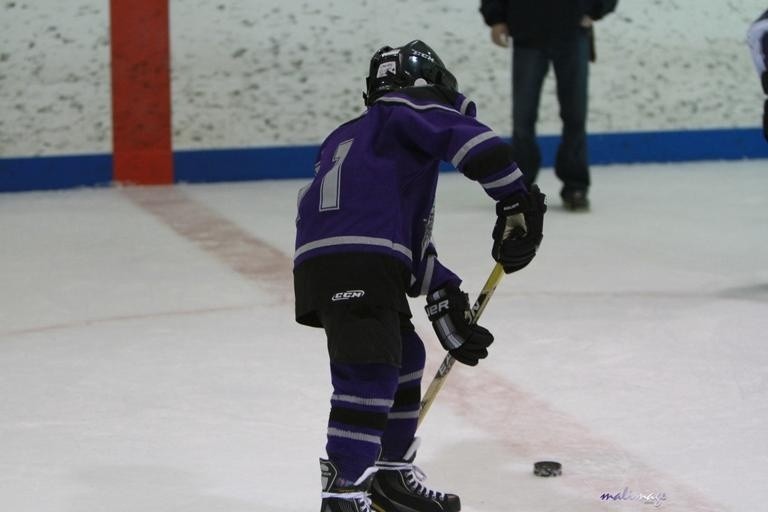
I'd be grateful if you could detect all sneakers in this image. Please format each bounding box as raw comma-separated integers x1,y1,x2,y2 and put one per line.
560,182,589,211
317,453,461,512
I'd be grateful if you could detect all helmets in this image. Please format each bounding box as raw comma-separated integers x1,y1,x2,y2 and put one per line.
366,38,455,106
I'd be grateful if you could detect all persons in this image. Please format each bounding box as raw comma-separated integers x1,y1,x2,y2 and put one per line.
477,0,619,212
290,37,544,512
744,7,767,147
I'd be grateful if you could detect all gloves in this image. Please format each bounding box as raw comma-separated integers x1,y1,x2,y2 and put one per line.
424,282,495,369
488,182,549,277
